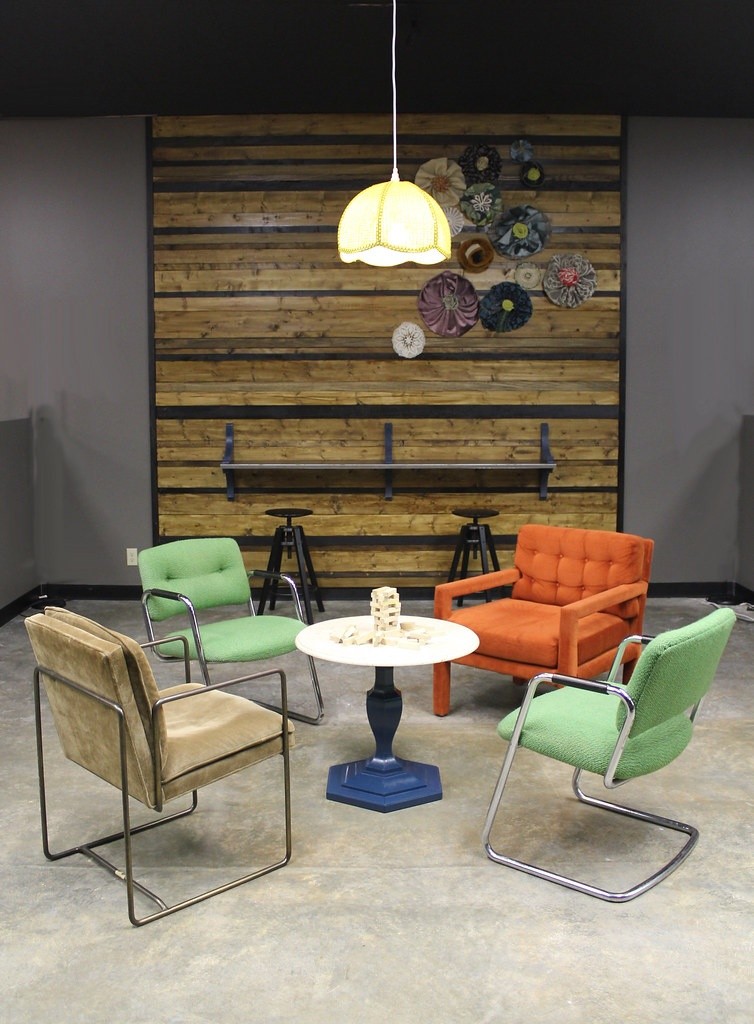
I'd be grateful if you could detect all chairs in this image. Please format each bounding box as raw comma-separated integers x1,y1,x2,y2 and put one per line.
136,536,324,725
434,539,655,717
481,605,738,904
25,605,295,927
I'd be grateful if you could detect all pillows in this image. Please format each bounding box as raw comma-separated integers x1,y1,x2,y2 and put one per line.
44,606,168,772
513,523,644,620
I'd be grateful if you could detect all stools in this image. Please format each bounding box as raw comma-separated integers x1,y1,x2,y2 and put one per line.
256,508,324,626
447,508,506,607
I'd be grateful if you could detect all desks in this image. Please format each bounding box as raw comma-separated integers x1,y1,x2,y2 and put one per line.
292,615,480,811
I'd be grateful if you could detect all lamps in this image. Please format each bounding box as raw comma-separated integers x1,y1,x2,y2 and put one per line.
337,0,451,268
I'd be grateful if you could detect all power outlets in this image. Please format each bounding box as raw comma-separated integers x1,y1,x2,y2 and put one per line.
126,547,138,567
